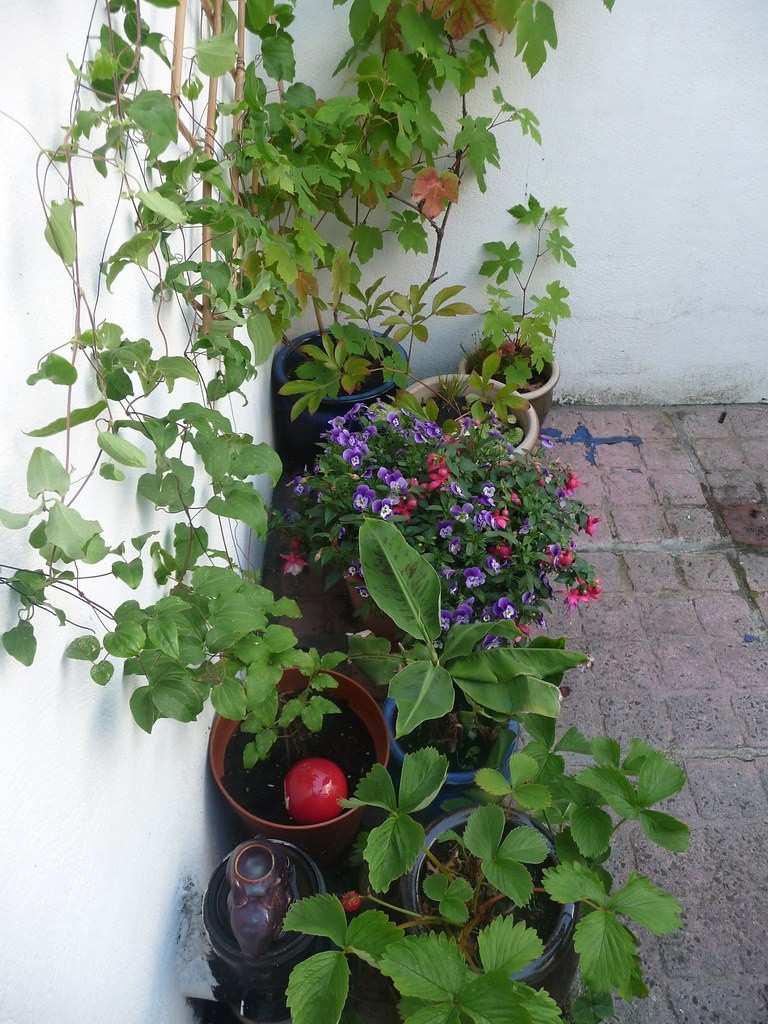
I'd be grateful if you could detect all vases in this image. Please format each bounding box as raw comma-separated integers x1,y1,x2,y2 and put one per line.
341,569,402,639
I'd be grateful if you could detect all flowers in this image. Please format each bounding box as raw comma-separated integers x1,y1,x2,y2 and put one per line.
280,395,602,646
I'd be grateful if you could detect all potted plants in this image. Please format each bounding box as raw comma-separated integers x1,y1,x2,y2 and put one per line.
1,0,693,1024
